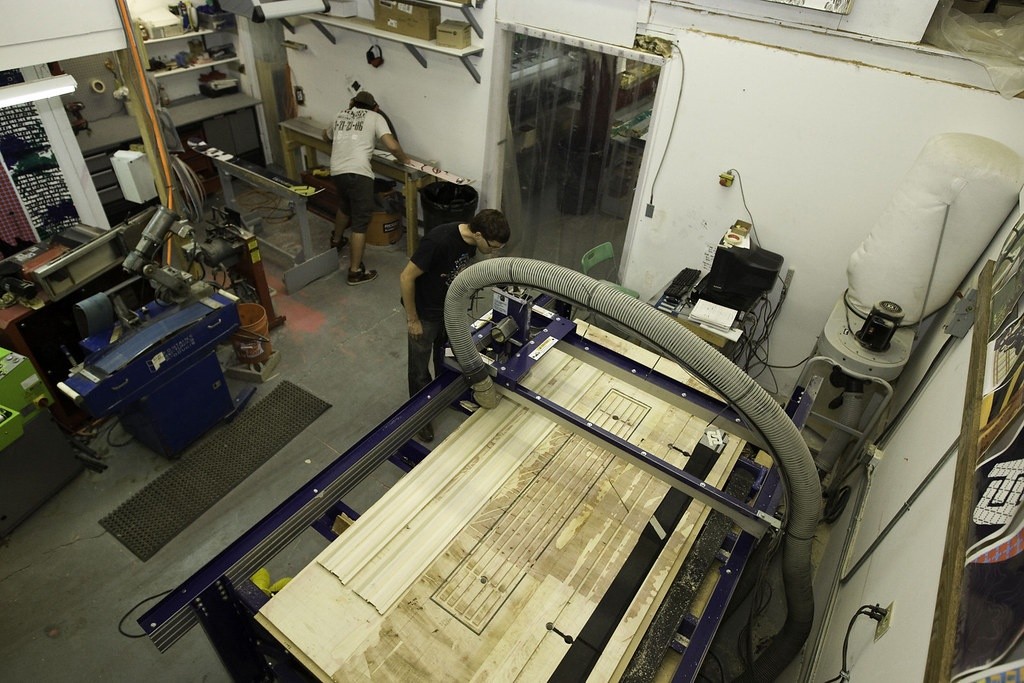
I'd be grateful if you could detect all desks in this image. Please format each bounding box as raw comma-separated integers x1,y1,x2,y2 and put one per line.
278,117,438,259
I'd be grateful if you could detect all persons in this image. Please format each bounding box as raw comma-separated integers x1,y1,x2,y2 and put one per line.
322,91,411,286
400,208,511,443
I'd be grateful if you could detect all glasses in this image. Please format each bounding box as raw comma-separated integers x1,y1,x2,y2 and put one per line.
486,239,507,251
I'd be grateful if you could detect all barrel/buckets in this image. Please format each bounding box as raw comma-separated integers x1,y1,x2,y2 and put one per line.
362,191,401,245
231,304,272,364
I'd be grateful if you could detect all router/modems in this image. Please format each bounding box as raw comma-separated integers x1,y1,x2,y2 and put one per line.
688,300,738,332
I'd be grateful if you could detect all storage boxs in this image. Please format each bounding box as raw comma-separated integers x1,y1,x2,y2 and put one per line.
436,19,471,49
374,0,441,41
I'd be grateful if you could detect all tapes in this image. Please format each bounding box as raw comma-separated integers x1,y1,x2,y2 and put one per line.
723,232,743,245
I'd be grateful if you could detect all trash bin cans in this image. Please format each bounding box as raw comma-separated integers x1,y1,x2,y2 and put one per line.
419,180,480,240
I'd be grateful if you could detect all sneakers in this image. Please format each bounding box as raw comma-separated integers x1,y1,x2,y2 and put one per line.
330,231,349,253
347,262,378,285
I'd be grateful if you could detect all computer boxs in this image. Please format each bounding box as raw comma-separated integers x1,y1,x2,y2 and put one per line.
691,272,763,322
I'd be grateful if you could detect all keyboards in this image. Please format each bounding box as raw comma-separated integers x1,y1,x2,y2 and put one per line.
664,267,701,299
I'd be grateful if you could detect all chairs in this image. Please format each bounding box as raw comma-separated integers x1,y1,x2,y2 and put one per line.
581,242,640,299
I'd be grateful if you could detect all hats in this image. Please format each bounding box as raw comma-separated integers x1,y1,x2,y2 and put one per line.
354,92,374,107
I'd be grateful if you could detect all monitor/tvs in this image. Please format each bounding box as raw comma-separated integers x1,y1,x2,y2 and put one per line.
707,228,784,299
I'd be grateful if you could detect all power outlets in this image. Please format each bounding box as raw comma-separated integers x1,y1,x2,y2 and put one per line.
874,601,894,643
645,203,655,218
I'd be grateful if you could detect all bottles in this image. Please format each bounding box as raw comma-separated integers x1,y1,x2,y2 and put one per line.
139,23,148,41
181,4,188,28
161,87,169,105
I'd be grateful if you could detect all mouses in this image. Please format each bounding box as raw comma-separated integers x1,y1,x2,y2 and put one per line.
666,298,679,304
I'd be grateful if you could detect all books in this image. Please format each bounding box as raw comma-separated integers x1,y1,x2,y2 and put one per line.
687,298,744,343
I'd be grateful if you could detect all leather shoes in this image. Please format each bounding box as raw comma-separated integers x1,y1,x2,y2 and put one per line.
417,423,434,442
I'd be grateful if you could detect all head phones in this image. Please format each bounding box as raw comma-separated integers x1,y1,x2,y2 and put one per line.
366,45,384,68
349,97,379,112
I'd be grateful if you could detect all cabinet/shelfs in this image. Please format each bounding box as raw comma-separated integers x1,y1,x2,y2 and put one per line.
76,28,264,206
277,0,483,84
